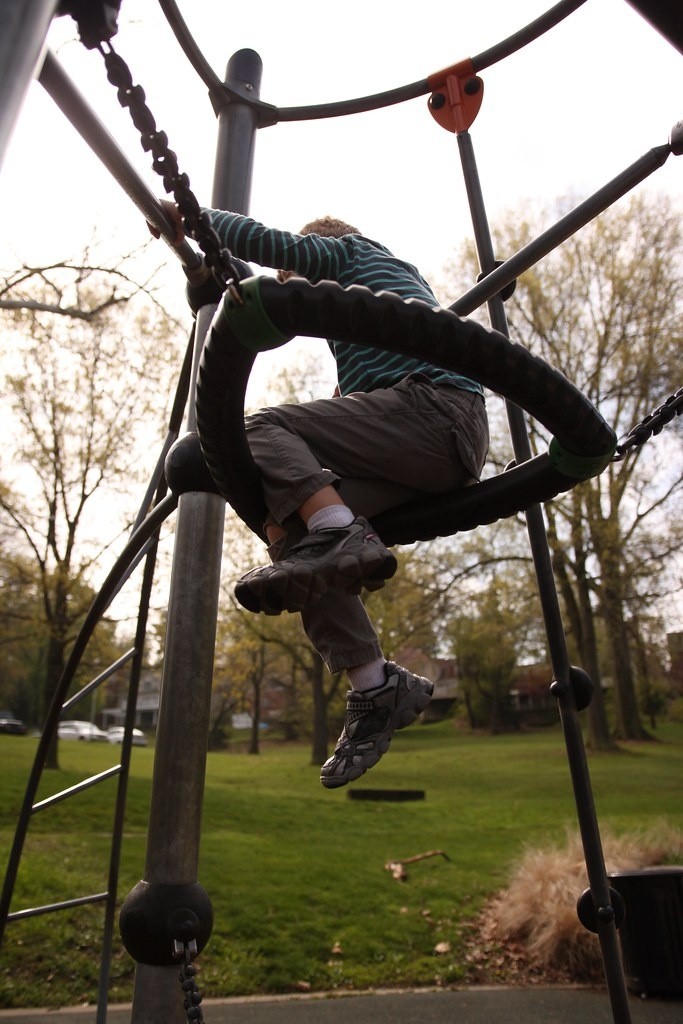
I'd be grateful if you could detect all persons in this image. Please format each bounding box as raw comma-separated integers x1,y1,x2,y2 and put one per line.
148,200,490,790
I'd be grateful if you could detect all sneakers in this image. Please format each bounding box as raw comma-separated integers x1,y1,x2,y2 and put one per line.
235,515,398,616
321,661,435,788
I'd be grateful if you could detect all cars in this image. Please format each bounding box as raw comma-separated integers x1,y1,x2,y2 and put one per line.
57,720,149,747
0,719,26,734
